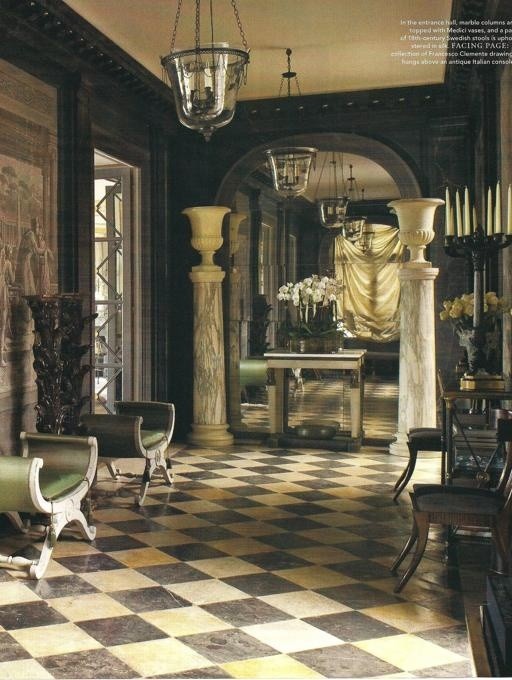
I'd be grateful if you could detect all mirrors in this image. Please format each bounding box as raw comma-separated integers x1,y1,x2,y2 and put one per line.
223,151,403,448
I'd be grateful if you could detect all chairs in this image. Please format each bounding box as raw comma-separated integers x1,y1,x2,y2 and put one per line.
392,426,440,501
390,442,512,593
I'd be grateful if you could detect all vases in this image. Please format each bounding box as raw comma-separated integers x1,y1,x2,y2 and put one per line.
290,339,336,350
462,338,490,372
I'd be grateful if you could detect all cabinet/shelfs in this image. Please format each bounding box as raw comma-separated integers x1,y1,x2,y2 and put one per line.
439,367,512,483
263,353,365,452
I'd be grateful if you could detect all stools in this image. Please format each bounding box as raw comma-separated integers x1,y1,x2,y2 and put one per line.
0,431,99,580
82,399,175,506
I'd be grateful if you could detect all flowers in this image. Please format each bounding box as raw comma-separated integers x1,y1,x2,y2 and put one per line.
274,273,344,339
438,290,512,335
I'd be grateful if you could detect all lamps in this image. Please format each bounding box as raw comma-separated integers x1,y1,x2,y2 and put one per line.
362,190,375,252
340,164,369,244
161,0,251,141
314,153,347,228
262,46,319,201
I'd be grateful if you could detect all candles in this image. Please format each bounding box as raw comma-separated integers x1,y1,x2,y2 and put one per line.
439,181,512,237
